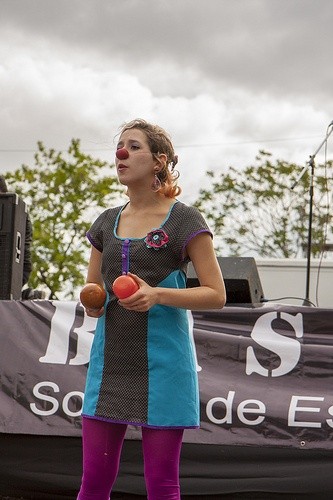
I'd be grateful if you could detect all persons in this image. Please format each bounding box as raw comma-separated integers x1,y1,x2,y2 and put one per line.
76,119,226,500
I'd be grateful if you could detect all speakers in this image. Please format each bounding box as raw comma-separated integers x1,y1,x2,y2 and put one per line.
186,257,265,308
0,192,27,301
